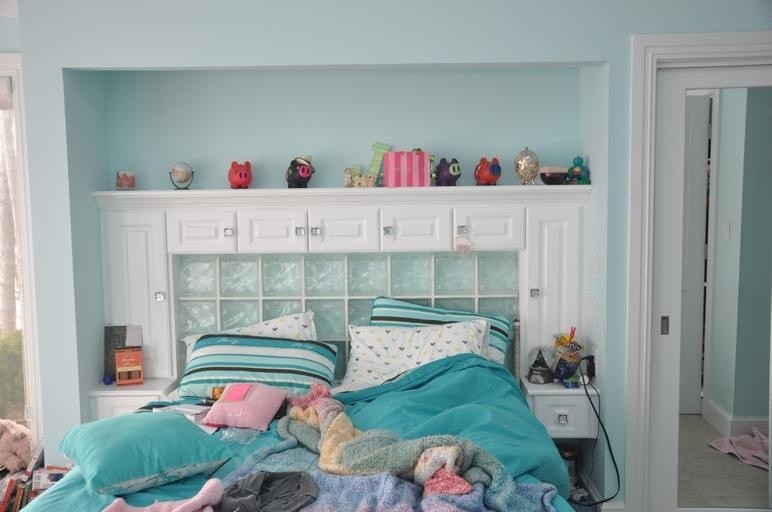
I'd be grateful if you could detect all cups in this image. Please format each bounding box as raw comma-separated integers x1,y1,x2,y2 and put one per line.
115,170,136,191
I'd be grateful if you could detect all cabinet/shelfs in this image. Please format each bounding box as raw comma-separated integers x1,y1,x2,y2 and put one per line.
165,185,525,252
518,186,593,375
93,189,181,378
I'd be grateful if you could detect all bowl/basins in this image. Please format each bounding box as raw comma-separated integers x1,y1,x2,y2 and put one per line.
540,166,568,185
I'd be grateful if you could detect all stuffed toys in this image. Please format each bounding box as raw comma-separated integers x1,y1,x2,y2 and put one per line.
285,157,317,189
567,154,591,183
434,157,462,187
227,159,253,190
474,156,501,185
1,419,32,474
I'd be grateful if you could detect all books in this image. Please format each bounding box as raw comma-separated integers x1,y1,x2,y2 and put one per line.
113,344,145,386
0,464,69,512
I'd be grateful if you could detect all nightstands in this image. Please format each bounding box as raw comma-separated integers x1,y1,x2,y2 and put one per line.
521,375,598,440
87,379,174,420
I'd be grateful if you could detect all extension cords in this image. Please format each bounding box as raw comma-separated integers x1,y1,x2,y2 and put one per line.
569,487,589,502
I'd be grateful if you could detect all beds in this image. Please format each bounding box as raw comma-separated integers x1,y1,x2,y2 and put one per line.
18,355,575,512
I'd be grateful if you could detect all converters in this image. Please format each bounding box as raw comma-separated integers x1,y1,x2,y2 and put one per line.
587,355,595,377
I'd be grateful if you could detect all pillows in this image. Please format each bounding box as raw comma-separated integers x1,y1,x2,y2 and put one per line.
181,334,339,399
370,298,511,366
182,310,318,361
58,412,232,495
340,319,490,393
